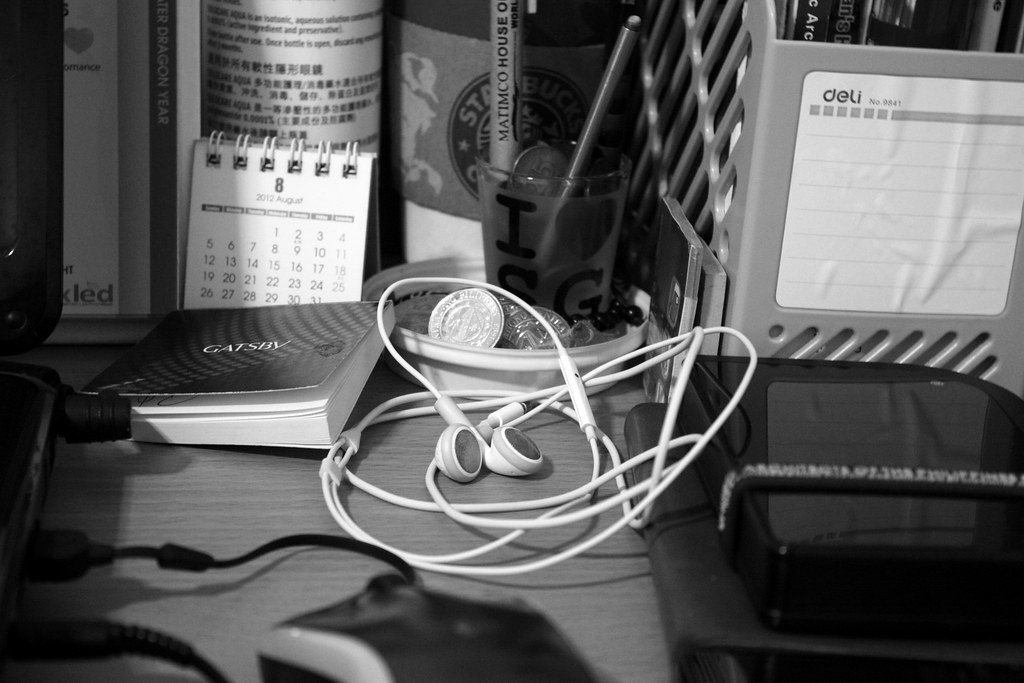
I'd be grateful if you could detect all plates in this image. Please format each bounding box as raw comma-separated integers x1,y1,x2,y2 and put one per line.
363,257,648,401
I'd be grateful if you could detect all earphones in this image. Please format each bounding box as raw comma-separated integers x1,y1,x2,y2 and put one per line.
433,426,481,483
436,395,544,477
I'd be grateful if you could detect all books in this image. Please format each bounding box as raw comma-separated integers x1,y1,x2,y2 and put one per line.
776,0,867,44
720,0,1024,215
45,0,396,445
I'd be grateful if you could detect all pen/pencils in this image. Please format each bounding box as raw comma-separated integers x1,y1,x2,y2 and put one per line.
530,15,642,270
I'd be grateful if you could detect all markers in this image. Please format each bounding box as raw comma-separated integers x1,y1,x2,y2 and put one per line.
487,0,530,176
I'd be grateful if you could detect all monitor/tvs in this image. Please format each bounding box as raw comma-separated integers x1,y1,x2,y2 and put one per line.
0,0,65,358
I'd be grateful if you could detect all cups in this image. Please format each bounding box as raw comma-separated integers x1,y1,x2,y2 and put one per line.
475,146,632,318
